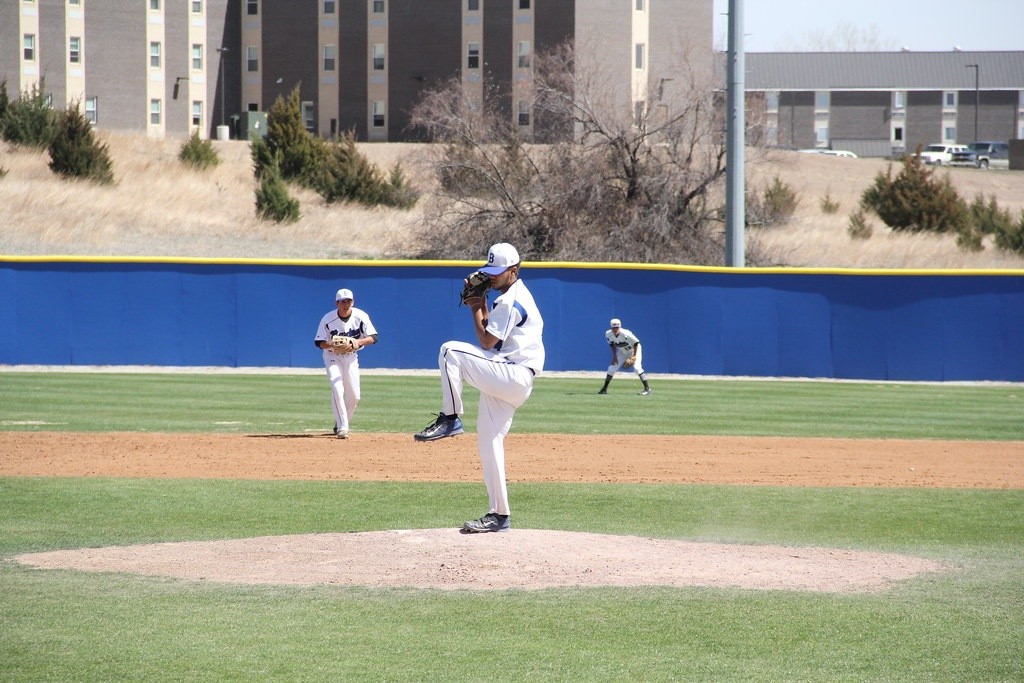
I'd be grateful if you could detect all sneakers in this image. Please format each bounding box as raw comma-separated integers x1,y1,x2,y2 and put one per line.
415,412,464,442
465,513,510,532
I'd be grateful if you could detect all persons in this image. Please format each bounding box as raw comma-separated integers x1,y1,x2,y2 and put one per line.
414,243,545,532
315,288,379,438
598,319,651,395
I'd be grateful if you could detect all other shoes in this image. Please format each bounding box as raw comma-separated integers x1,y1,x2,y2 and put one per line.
598,390,607,394
637,388,652,396
333,427,338,434
337,430,349,438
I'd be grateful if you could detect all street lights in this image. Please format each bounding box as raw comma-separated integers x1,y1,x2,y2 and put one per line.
964,65,979,143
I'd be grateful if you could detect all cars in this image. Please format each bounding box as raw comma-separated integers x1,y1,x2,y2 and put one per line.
798,148,858,159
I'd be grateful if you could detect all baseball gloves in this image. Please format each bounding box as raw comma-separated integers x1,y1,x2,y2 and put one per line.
331,335,360,356
461,270,492,306
622,355,637,369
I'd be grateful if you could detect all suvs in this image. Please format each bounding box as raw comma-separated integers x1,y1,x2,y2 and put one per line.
950,141,1010,171
910,143,969,167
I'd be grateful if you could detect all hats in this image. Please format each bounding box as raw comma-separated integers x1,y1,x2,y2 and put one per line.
610,319,621,328
477,242,520,275
335,289,354,301
